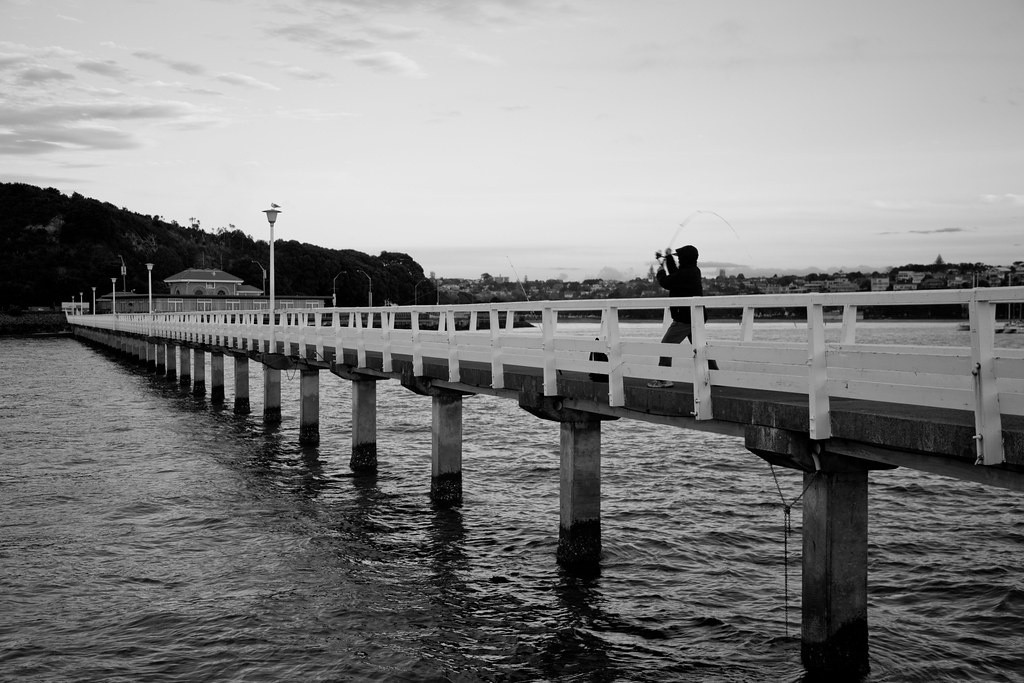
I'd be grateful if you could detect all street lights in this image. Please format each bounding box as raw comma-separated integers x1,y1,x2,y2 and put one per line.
91,287,96,326
262,209,280,354
80,292,83,325
429,278,439,304
145,263,154,336
252,261,265,297
357,270,371,306
111,278,117,330
415,280,426,305
72,295,74,321
333,271,347,307
118,255,125,295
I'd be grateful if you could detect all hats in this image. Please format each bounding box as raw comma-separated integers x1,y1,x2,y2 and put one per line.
672,245,699,261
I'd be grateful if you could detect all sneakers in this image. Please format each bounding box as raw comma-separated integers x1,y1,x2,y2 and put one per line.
646,378,675,389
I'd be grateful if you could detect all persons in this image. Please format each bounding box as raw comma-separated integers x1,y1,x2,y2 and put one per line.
647,245,719,387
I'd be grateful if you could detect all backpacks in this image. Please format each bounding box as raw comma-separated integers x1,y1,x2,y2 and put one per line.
587,337,609,382
